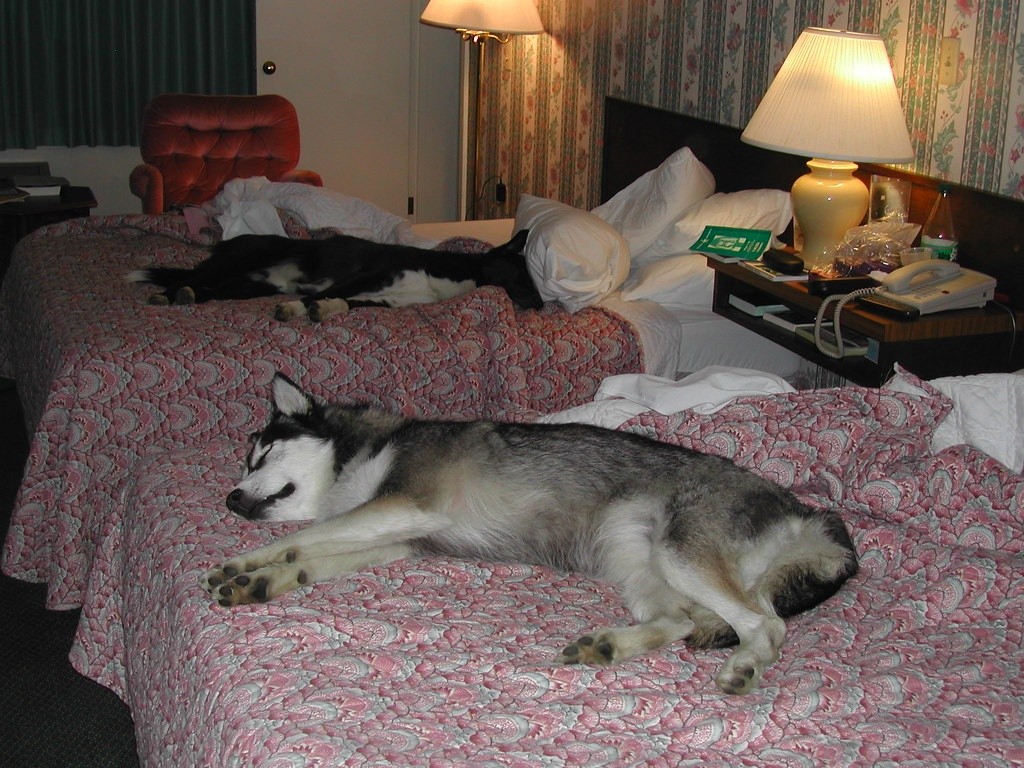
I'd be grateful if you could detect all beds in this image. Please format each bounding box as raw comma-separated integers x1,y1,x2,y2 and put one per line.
0,95,801,612
68,359,1024,768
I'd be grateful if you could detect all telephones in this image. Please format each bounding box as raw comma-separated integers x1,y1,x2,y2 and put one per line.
875,258,998,316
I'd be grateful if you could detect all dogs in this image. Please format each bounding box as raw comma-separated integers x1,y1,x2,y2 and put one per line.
120,230,543,322
198,370,861,695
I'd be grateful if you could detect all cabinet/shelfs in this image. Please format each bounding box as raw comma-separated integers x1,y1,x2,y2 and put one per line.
0,161,97,289
702,252,1022,384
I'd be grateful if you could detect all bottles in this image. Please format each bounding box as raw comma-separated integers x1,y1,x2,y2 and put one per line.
921,181,957,264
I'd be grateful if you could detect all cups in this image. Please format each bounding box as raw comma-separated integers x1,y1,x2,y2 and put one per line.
898,247,932,268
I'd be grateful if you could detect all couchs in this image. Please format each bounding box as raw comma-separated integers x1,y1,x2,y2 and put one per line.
130,94,324,218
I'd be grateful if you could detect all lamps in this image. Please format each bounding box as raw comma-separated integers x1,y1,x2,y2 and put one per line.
420,0,546,44
738,25,915,271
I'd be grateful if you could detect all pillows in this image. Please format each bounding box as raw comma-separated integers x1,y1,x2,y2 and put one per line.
631,188,793,272
590,146,717,263
515,192,632,312
620,252,716,306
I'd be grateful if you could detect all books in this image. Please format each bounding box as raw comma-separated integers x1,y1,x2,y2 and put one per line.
0,177,61,205
746,262,809,282
688,224,773,264
